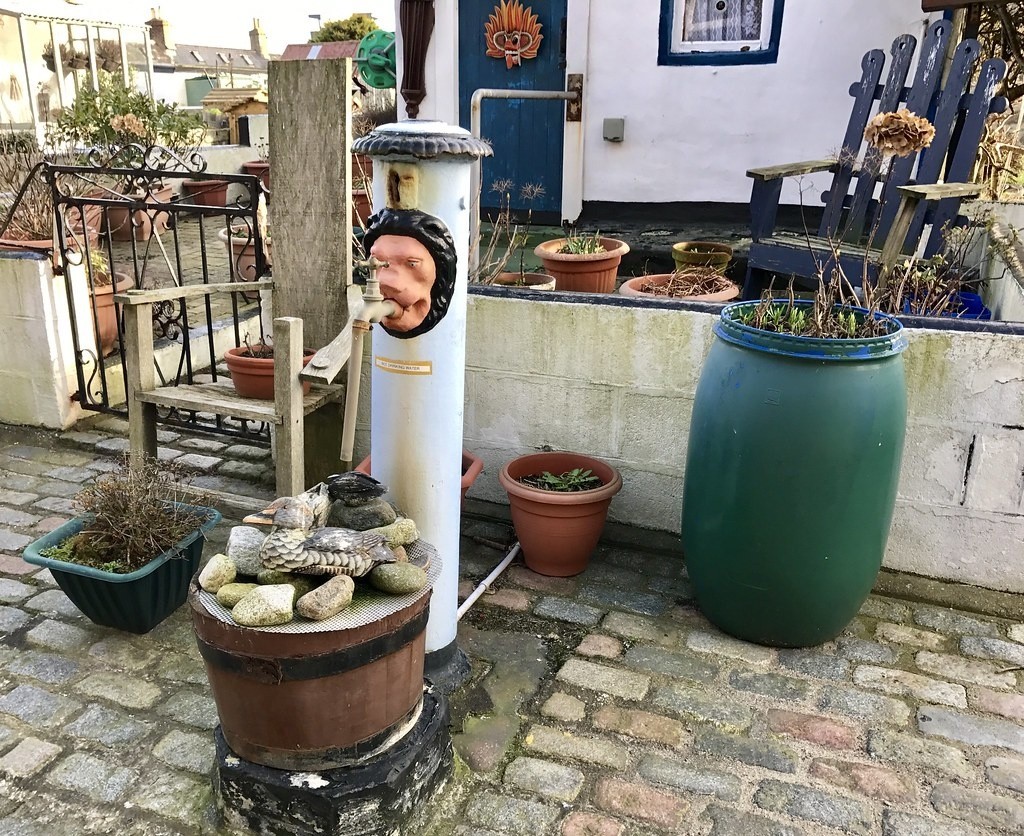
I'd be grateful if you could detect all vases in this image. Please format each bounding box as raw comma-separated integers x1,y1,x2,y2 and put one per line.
681,297,911,650
355,442,482,515
500,449,622,579
350,148,373,229
21,494,221,636
185,558,435,769
0,117,270,250
904,284,991,323
225,340,318,401
481,234,741,304
215,222,275,302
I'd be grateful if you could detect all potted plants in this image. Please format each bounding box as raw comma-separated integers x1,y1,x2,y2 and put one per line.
41,38,123,73
0,127,98,270
70,244,134,360
54,69,208,240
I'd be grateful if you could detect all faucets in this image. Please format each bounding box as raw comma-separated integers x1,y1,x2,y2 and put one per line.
337,254,395,332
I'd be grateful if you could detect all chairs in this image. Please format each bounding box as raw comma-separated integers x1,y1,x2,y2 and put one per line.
744,19,1010,310
113,56,369,518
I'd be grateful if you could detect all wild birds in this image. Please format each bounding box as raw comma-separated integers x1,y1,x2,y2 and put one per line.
244,497,398,578
